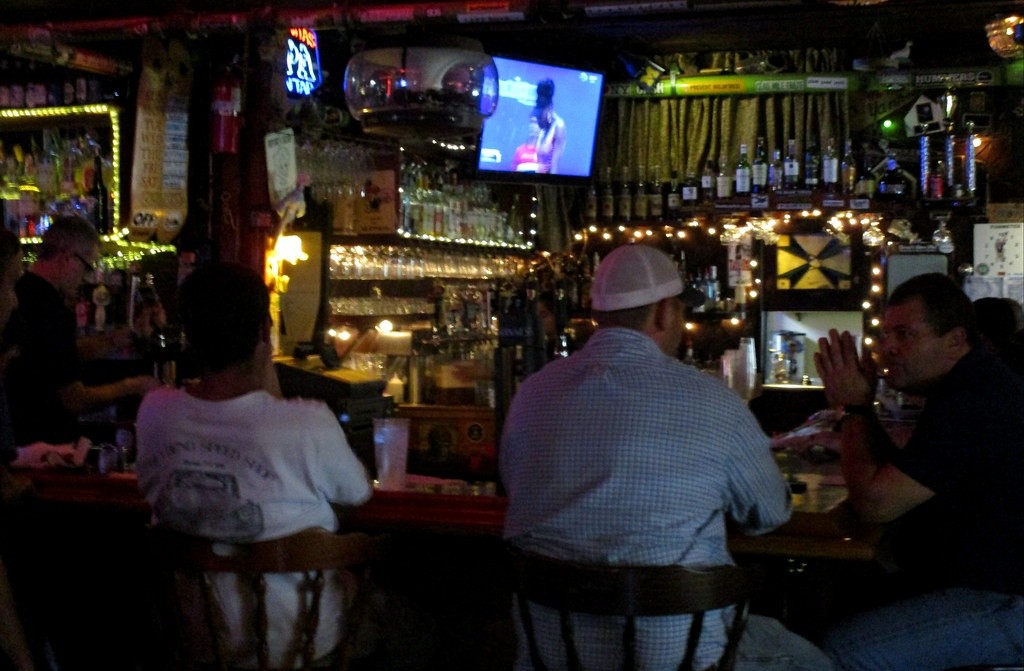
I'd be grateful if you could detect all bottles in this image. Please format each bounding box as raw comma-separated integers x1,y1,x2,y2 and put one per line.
769,151,782,190
399,166,524,243
88,153,111,236
822,138,840,195
582,166,614,225
805,136,819,191
633,165,649,222
842,138,877,199
784,138,801,188
665,171,680,220
928,151,946,199
702,160,717,204
706,265,721,301
880,153,905,193
735,144,751,195
717,156,733,204
649,165,663,221
752,137,768,193
682,171,697,207
616,167,633,222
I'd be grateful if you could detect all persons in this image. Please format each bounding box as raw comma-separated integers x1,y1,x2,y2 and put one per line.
136,262,427,671
496,242,836,671
972,297,1024,380
3,212,170,670
815,273,1024,671
511,106,540,171
0,224,28,670
536,98,567,173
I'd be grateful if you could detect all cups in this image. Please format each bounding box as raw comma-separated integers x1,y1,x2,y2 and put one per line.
372,417,409,490
738,336,758,377
719,351,749,399
334,250,517,278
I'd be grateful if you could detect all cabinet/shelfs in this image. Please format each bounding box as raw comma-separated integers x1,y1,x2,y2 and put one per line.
321,237,538,365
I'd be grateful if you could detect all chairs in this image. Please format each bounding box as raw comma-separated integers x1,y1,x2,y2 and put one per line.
505,547,767,671
152,526,381,671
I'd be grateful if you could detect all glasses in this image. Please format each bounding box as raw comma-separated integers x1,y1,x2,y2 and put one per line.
75,251,93,273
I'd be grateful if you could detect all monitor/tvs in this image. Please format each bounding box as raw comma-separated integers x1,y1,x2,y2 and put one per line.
475,56,607,186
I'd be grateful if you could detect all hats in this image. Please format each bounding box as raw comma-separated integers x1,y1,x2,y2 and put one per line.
530,105,551,116
591,241,700,311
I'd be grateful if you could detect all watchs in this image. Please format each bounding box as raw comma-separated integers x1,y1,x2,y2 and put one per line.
831,403,866,429
